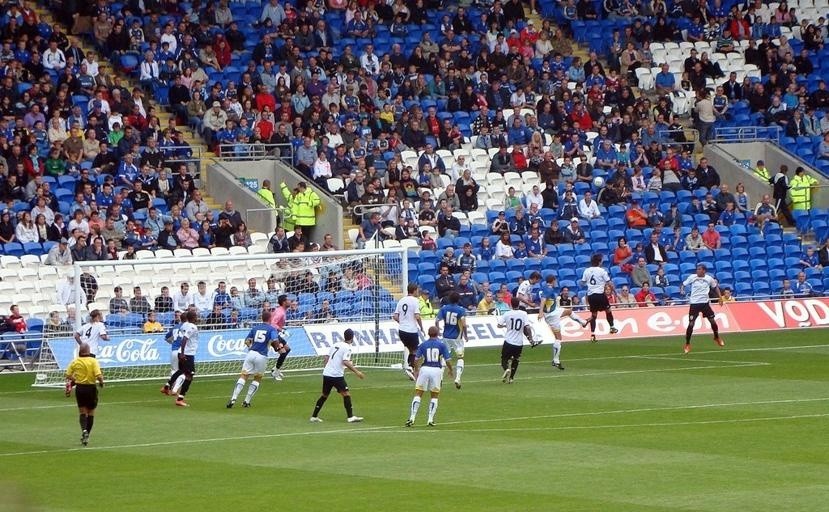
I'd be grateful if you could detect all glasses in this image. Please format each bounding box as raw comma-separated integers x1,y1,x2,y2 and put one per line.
622,289,628,292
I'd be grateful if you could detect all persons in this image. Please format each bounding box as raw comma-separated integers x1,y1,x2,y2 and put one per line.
280,177,320,252
269,295,294,382
734,183,751,213
400,199,419,222
559,286,572,308
436,293,469,390
498,283,513,306
227,312,282,409
161,0,221,215
583,254,619,342
720,203,736,225
396,217,409,242
492,210,511,234
197,282,213,324
215,213,236,247
111,285,128,316
223,1,320,177
526,186,544,209
461,2,543,173
645,168,663,190
46,2,159,222
66,229,82,249
686,227,703,250
205,210,215,229
279,189,300,236
341,270,357,292
505,186,524,210
420,201,438,226
543,0,631,184
479,236,494,261
316,299,334,323
632,257,652,287
157,279,174,317
682,167,699,191
283,271,302,301
123,220,141,244
789,166,818,209
680,264,728,351
613,237,633,267
45,237,73,266
511,210,529,237
166,309,189,394
517,272,541,313
264,279,280,301
356,213,397,252
219,201,243,226
206,307,227,328
497,300,534,384
629,165,645,191
326,267,338,291
158,218,181,248
632,243,646,264
69,236,90,260
779,278,795,298
560,191,582,220
800,247,818,270
527,229,547,256
646,204,664,229
769,166,791,225
44,311,73,337
603,282,618,306
295,241,314,269
792,272,814,298
720,287,735,302
433,265,455,297
755,194,778,226
687,197,704,216
438,206,461,241
696,157,723,187
617,285,637,308
162,312,198,408
543,178,560,211
407,328,454,429
80,266,98,310
124,245,140,260
701,192,717,212
302,272,319,291
443,248,458,273
477,293,495,316
229,286,244,314
199,220,216,249
1,2,45,247
176,217,200,249
561,182,578,204
538,275,593,373
75,310,108,362
545,220,567,246
459,242,476,271
214,281,230,310
355,0,397,211
102,239,120,260
398,3,459,202
458,188,480,212
84,237,109,260
636,283,658,306
646,233,669,264
100,220,125,244
655,268,669,287
86,224,105,245
312,330,367,422
695,0,829,158
659,159,682,191
703,222,721,250
176,279,195,319
6,305,29,351
246,278,264,309
320,1,353,216
394,284,423,379
436,199,450,216
627,200,649,230
287,225,312,252
144,312,163,333
131,285,151,312
494,232,512,260
716,185,736,212
257,180,281,234
707,278,723,302
455,170,478,193
268,226,290,253
271,258,290,284
307,243,321,272
819,238,829,267
406,218,422,240
617,177,633,203
495,291,509,312
598,179,620,207
233,221,252,248
417,290,436,319
524,203,547,229
422,230,437,249
319,234,343,279
64,346,106,447
668,227,688,251
142,227,159,250
753,160,773,184
515,240,529,259
563,216,586,243
287,249,303,269
454,276,480,310
579,190,601,219
633,1,694,165
49,213,67,240
667,202,682,228
478,281,493,307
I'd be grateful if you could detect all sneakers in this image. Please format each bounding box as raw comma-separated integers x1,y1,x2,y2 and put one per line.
346,416,365,423
241,400,251,408
683,343,692,352
582,317,593,328
454,379,461,390
160,387,171,395
609,327,618,334
551,360,565,370
271,366,285,381
590,334,599,344
309,417,323,423
175,399,191,407
427,422,437,427
226,399,235,408
404,419,414,427
80,429,89,447
500,369,511,384
531,340,543,349
713,336,725,346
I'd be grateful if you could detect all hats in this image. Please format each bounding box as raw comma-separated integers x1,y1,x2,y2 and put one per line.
498,211,505,216
79,342,91,355
212,101,221,107
445,247,455,254
164,220,174,225
570,216,579,223
464,242,471,246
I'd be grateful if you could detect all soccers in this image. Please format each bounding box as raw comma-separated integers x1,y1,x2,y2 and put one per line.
594,177,605,187
